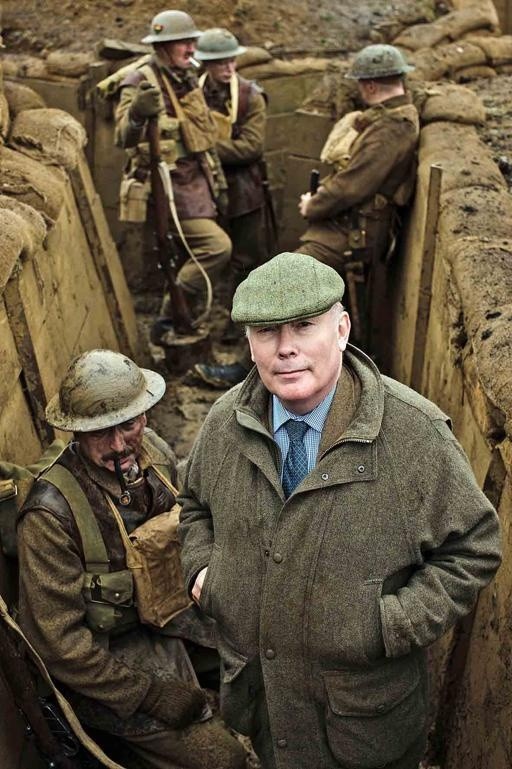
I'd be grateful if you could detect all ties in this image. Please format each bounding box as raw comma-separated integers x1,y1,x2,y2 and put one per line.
279,420,313,501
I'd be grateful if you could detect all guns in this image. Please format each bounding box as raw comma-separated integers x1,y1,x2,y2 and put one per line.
148,88,198,335
0,616,81,768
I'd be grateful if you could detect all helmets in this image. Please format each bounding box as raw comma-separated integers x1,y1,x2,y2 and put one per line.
139,9,201,45
342,43,416,82
45,348,167,434
193,27,248,61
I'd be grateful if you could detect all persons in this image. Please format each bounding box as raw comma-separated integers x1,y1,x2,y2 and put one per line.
191,42,421,391
196,25,274,345
180,250,504,769
10,350,244,769
109,10,235,375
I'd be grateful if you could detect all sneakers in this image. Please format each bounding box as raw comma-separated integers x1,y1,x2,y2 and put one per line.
193,361,247,390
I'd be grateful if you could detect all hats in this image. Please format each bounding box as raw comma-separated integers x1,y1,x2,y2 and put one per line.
229,253,345,327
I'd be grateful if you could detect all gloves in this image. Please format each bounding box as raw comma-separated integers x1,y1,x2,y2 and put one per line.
146,679,209,731
131,79,166,120
